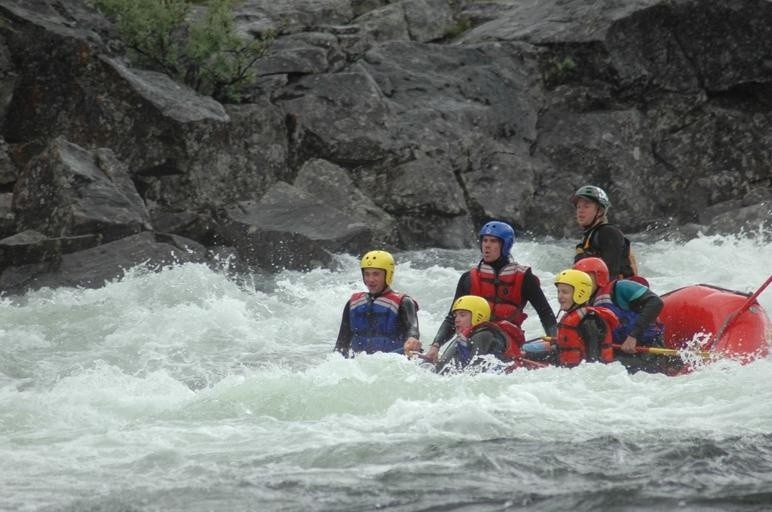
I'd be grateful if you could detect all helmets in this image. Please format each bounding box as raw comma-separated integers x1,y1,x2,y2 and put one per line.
575,185,610,211
480,221,515,256
361,250,395,285
452,295,491,326
554,257,609,305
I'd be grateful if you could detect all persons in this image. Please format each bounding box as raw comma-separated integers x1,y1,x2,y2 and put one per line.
334,250,421,359
567,185,637,283
437,294,510,378
553,268,620,368
571,256,669,374
425,221,558,369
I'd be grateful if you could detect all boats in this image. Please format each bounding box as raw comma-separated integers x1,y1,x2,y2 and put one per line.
517,285,772,375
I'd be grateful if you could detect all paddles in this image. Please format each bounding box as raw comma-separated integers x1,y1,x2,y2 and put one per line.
543,336,710,358
